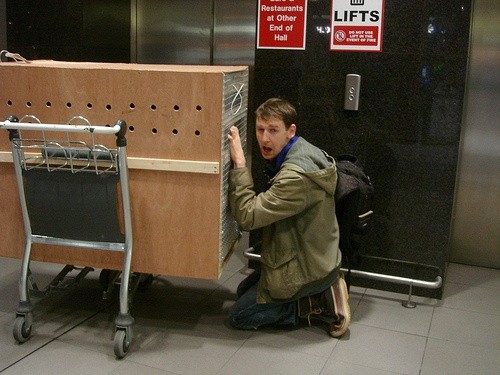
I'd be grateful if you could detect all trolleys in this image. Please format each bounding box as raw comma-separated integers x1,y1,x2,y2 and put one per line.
0,114,154,358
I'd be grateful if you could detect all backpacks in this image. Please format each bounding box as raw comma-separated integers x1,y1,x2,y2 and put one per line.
336,160,374,250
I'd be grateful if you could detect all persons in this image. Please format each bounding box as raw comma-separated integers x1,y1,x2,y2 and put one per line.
228,98,351,337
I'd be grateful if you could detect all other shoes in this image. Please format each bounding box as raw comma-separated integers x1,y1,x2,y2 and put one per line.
297,272,352,337
